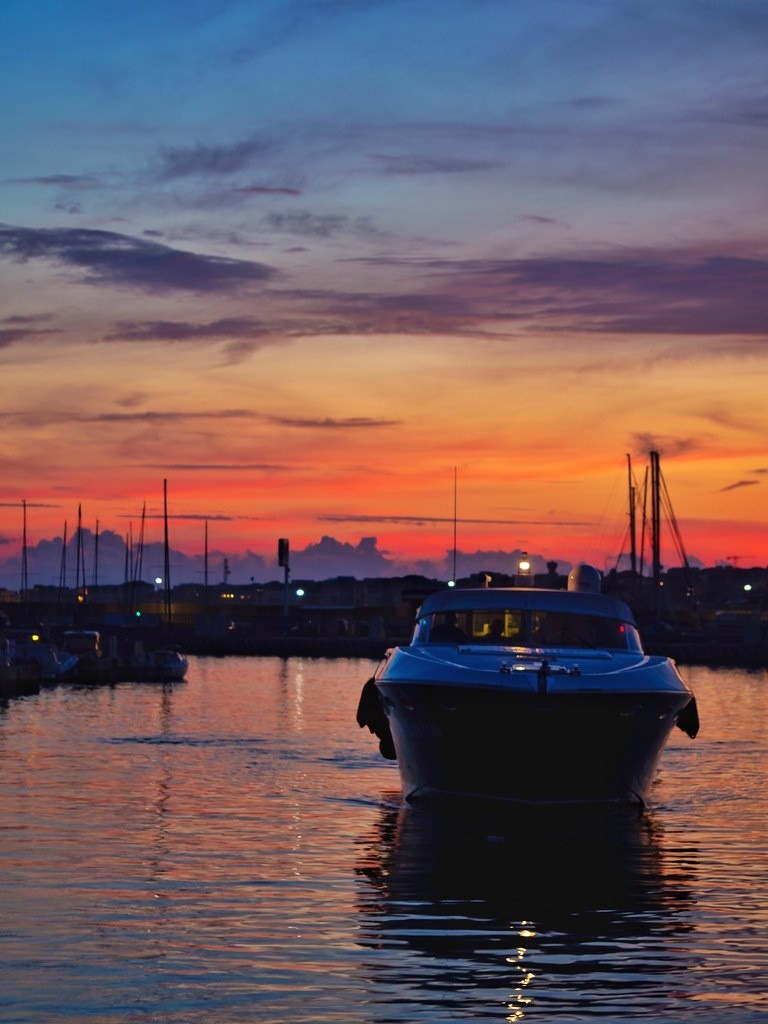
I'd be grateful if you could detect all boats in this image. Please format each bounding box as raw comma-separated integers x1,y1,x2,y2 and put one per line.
357,586,700,821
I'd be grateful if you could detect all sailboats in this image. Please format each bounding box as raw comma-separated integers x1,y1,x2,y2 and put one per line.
0,479,188,689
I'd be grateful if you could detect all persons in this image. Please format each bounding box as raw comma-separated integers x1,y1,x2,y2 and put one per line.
430,612,464,641
485,620,505,640
